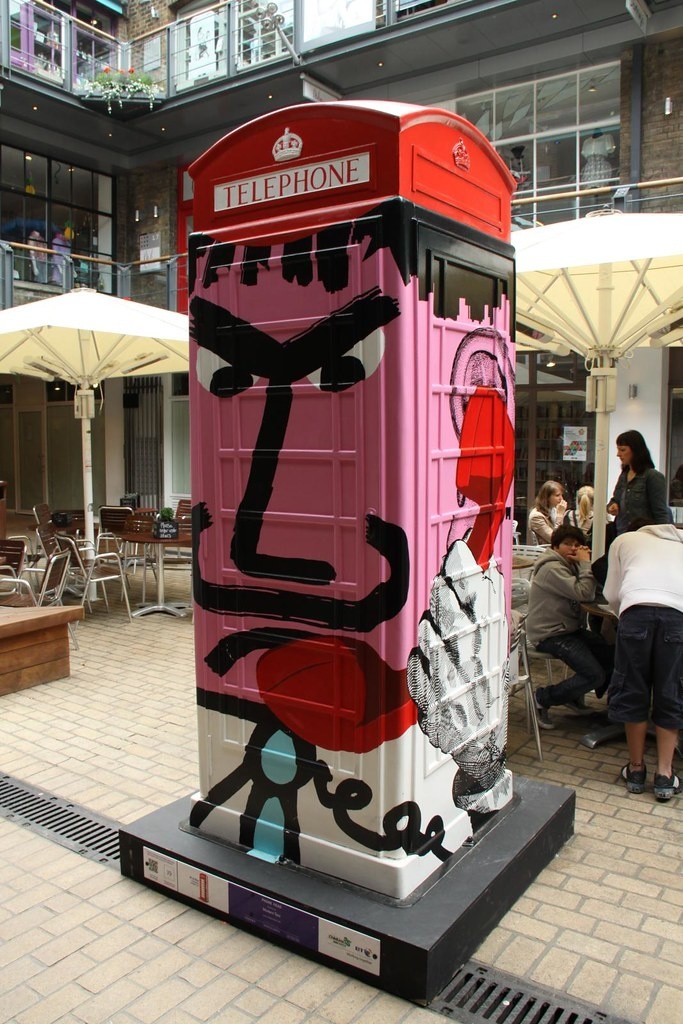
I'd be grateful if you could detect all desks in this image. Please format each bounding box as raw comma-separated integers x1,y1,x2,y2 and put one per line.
580,596,683,758
512,555,535,570
27,522,99,599
121,533,192,619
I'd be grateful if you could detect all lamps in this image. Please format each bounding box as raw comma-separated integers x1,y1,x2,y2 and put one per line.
628,383,637,400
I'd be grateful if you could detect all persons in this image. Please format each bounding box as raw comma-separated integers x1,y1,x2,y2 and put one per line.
27,230,76,287
607,429,671,540
529,480,568,551
603,522,683,800
581,128,616,189
525,524,613,729
562,486,615,555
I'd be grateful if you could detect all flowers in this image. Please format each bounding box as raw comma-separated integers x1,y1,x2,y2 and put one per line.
85,68,165,114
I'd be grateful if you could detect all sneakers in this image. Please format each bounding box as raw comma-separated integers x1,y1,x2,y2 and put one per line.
654,769,683,798
564,699,594,714
533,691,555,729
622,762,648,794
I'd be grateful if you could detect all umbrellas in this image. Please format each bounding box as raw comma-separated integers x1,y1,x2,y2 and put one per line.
0,287,189,600
511,208,683,562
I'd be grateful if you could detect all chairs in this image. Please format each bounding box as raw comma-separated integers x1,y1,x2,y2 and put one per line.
0,539,27,595
36,519,92,615
96,505,139,574
51,531,132,632
176,499,192,557
511,613,544,764
513,544,545,582
120,515,157,580
0,547,79,649
513,520,521,552
32,503,92,547
511,578,569,698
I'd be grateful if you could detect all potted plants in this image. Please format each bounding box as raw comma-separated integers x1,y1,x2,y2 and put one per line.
152,508,179,538
120,492,139,511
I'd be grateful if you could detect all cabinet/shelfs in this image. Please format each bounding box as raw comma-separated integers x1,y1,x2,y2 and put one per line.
515,416,594,514
